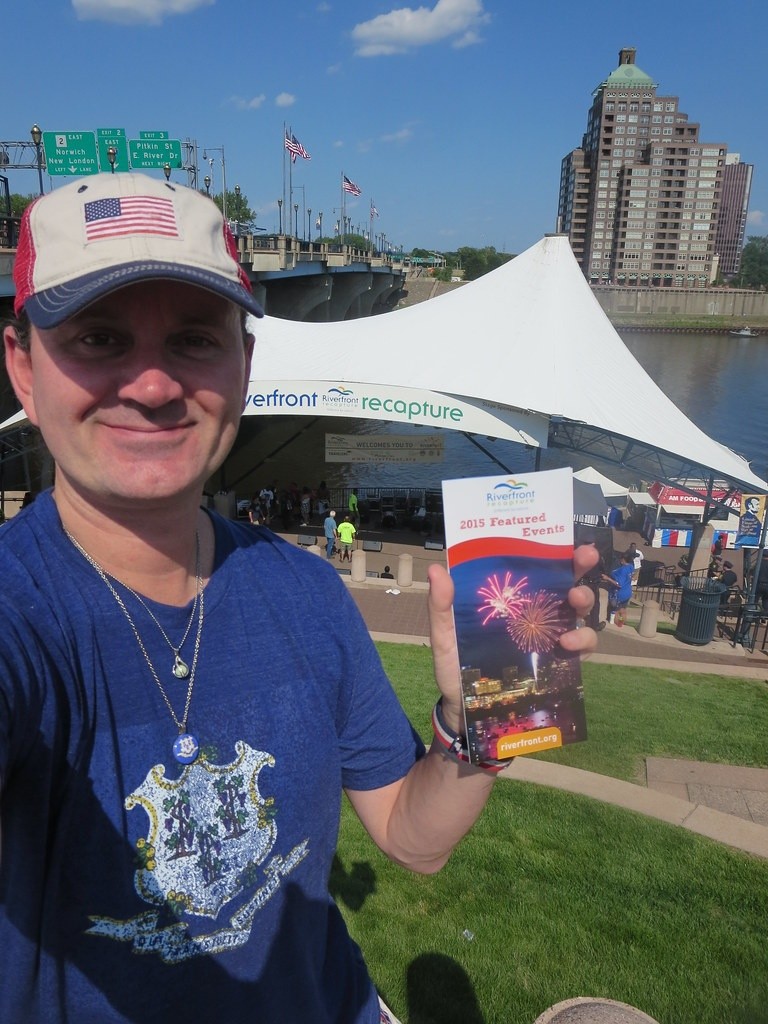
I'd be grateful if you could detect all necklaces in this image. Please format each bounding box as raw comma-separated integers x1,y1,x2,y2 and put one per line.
66,528,205,765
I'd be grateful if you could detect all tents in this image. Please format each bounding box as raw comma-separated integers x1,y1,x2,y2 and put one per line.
572,465,628,525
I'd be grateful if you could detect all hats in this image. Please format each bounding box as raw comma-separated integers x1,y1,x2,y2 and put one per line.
13,171,265,331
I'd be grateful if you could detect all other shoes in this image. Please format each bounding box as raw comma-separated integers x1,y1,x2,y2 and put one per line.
300,524,307,526
594,622,606,631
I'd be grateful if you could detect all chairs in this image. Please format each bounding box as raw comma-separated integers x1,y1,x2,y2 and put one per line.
368,492,421,514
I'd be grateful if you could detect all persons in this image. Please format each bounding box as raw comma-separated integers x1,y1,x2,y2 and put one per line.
236,485,278,525
324,511,356,563
381,566,394,579
0,171,589,1024
282,479,331,532
575,533,644,634
750,549,768,623
348,488,360,530
708,535,737,605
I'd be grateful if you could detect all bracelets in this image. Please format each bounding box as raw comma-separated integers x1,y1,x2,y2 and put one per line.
431,695,514,771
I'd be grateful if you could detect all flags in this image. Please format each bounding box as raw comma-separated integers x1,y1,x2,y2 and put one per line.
285,130,311,164
343,175,362,197
370,206,378,220
